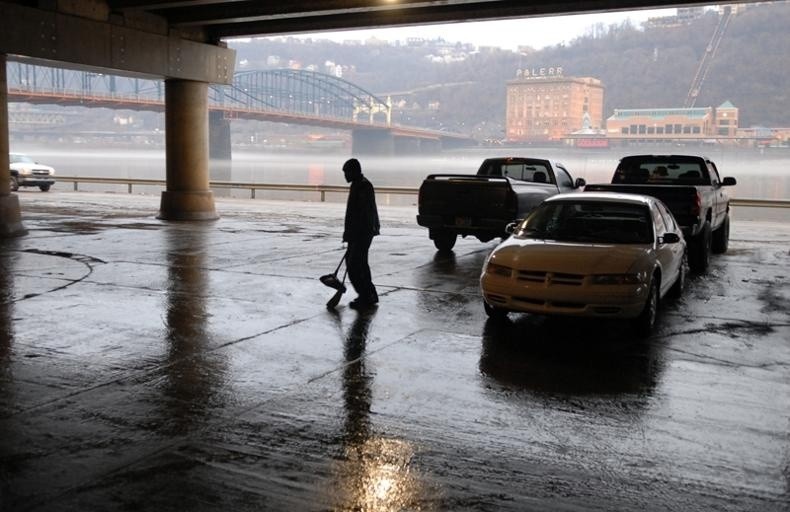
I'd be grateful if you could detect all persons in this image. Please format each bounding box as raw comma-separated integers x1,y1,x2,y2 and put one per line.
342,158,382,308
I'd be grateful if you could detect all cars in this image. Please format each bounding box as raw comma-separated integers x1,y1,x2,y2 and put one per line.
478,190,691,327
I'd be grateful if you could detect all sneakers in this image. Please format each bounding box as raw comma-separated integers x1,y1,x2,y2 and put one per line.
350,294,379,308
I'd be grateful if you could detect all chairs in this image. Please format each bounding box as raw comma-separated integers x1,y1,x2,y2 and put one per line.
534,171,547,183
641,168,700,179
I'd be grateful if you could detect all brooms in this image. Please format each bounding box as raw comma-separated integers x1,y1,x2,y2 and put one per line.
326,265,348,310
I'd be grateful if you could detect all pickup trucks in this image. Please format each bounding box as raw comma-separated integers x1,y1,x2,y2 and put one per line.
416,156,587,251
582,154,736,272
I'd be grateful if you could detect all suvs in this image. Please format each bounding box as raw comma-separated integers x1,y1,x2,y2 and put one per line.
9,152,57,193
